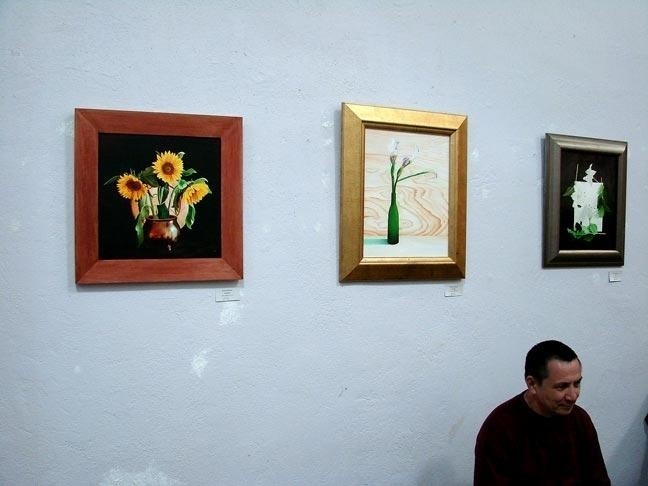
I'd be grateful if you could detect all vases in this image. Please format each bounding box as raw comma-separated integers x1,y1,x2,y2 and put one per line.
387,188,400,244
137,214,185,254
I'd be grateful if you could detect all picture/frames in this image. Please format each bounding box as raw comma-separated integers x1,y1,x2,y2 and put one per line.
337,97,468,286
541,131,629,271
73,104,245,288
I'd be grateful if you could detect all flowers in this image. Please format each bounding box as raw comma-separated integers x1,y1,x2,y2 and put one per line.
385,139,439,195
103,142,213,245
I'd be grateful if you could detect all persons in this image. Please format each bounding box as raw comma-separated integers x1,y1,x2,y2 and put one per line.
475,341,612,486
